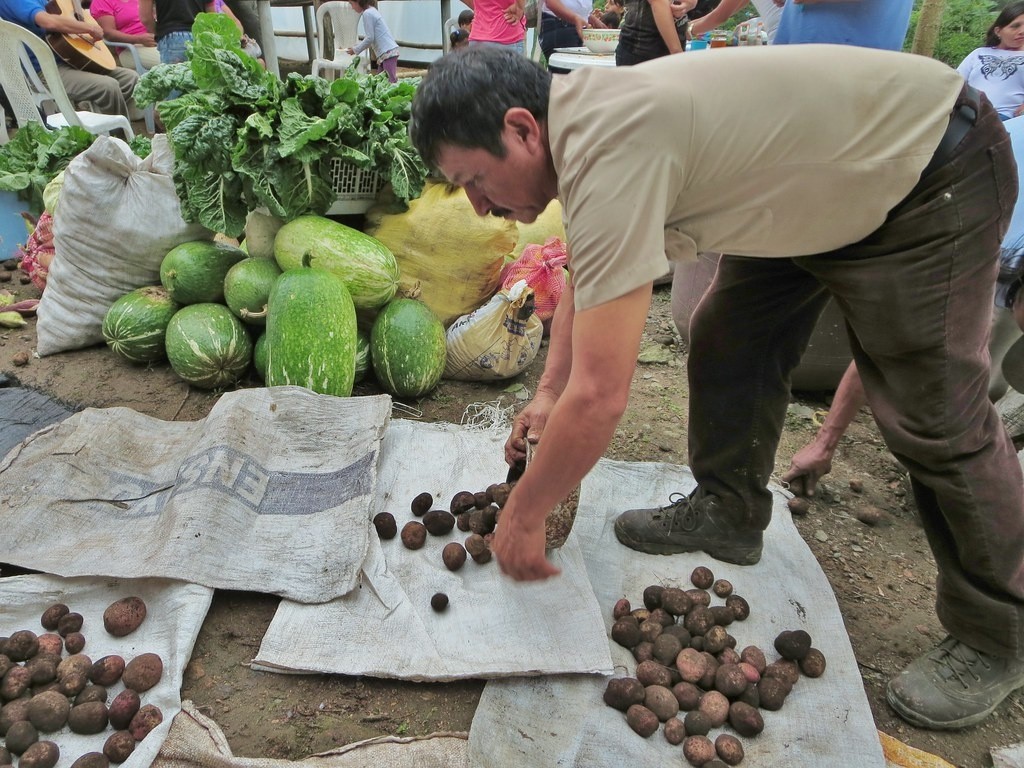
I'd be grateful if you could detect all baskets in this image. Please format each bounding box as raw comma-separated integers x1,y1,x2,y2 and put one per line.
254,156,387,216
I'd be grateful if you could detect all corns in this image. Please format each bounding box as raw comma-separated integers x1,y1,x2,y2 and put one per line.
0,210,56,326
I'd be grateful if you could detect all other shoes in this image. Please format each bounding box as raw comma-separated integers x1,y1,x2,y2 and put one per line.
886,634,1024,731
614,484,763,566
153,110,164,134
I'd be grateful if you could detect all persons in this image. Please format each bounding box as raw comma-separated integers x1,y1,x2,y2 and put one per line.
466,0,527,58
346,0,400,83
537,0,786,72
772,0,915,54
955,1,1024,121
0,0,264,151
774,113,1024,498
458,9,474,33
449,29,469,48
410,43,1024,731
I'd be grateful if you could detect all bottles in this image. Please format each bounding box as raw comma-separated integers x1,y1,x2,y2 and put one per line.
711,32,727,48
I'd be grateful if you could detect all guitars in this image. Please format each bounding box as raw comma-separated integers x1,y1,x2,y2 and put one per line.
44,0,117,74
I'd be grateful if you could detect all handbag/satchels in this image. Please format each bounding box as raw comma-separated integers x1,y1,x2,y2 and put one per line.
244,33,262,57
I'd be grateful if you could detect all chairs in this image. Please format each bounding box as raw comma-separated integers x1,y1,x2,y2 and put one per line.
312,2,370,83
445,17,462,54
0,18,156,145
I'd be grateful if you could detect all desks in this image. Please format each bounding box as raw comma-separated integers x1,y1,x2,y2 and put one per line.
547,51,618,69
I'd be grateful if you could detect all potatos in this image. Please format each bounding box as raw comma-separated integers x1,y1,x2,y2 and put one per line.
373,480,517,571
0,596,163,768
431,592,449,609
785,476,880,527
12,352,29,366
604,566,827,768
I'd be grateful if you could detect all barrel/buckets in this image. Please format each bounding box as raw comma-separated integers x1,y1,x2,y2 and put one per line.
691,40,707,51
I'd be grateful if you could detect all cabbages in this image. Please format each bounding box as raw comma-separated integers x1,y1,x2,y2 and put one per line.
42,173,61,215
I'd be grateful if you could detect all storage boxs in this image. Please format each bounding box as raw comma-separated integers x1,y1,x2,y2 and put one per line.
246,156,387,217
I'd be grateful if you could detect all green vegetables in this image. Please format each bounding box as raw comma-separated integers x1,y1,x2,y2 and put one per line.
131,12,429,236
0,119,151,191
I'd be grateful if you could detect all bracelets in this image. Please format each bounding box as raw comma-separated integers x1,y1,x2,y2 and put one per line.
687,23,694,40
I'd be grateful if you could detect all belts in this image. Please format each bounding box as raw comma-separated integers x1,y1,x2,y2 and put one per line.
921,86,981,177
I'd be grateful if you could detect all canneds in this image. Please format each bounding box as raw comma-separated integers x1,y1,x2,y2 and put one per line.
711,34,727,48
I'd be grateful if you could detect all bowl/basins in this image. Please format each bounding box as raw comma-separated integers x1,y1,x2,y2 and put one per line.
581,28,622,53
505,438,582,549
